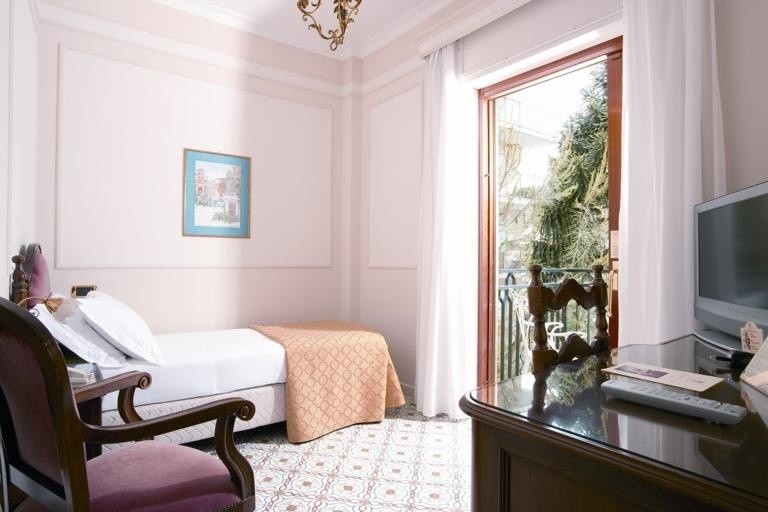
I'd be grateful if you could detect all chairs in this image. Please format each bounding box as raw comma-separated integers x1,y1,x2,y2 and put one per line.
1,296,256,512
527,264,612,372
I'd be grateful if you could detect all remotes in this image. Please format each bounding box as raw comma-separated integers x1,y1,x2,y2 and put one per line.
599,375,749,429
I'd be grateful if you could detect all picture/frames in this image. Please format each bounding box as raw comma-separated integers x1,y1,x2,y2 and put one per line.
182,149,252,238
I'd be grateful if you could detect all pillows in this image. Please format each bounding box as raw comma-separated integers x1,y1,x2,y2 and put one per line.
29,290,163,368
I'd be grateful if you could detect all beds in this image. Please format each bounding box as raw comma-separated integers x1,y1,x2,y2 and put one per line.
12,243,385,454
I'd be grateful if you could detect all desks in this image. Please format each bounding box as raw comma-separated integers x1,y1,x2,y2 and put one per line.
458,331,768,512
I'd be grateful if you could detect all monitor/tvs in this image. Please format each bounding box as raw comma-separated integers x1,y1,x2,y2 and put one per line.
692,338,768,497
690,179,768,353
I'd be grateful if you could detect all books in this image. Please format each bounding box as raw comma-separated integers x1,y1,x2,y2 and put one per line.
598,361,726,399
